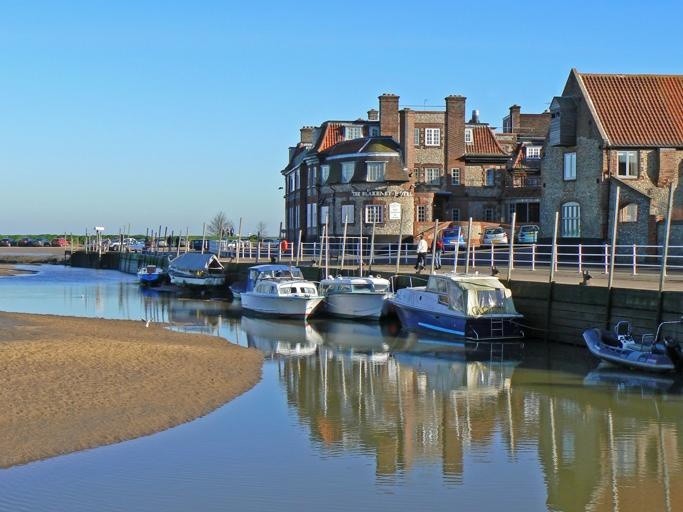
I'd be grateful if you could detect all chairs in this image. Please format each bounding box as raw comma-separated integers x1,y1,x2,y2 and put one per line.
629,334,655,353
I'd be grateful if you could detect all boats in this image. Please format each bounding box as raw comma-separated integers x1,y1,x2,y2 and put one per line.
230,264,305,298
168,253,226,293
136,263,167,285
582,326,676,373
386,272,525,343
318,273,392,322
241,275,323,321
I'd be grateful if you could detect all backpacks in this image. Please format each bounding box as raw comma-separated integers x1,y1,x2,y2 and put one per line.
436,240,443,249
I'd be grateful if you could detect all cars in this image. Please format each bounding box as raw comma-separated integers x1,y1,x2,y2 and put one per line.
87,236,280,252
431,227,468,254
0,237,70,247
478,227,509,248
518,225,544,244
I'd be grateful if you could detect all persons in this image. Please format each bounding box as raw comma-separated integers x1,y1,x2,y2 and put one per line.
311,260,317,267
167,235,174,252
414,236,428,271
328,271,343,289
222,227,234,238
430,235,445,270
103,237,112,251
176,236,179,250
